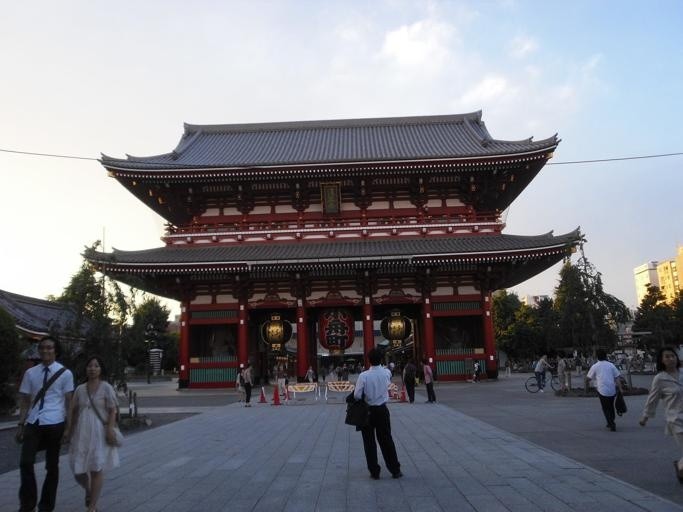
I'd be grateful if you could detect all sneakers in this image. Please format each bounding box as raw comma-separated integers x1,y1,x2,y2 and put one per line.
370,470,380,479
673,460,683,484
391,471,403,479
606,424,616,431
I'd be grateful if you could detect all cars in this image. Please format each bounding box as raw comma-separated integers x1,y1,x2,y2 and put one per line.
607,350,645,370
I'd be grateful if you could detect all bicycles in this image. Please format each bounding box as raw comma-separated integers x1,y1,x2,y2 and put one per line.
523,364,563,394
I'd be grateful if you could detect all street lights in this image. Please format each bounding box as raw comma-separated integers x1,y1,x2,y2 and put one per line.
143,322,158,383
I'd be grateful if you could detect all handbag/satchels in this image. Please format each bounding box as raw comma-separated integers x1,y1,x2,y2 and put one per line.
104,423,123,447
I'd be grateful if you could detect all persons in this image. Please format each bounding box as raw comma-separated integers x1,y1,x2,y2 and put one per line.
60,352,120,512
637,346,683,485
14,336,73,511
472,360,481,384
556,353,568,393
352,348,403,478
583,350,622,431
534,355,549,392
504,351,645,376
234,358,436,406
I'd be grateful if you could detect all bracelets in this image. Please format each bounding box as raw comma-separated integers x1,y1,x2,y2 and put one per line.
17,422,25,426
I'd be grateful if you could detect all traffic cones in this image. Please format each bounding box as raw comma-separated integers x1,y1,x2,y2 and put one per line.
388,382,407,402
258,383,292,407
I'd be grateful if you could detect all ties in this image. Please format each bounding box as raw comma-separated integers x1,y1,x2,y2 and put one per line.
39,367,49,411
